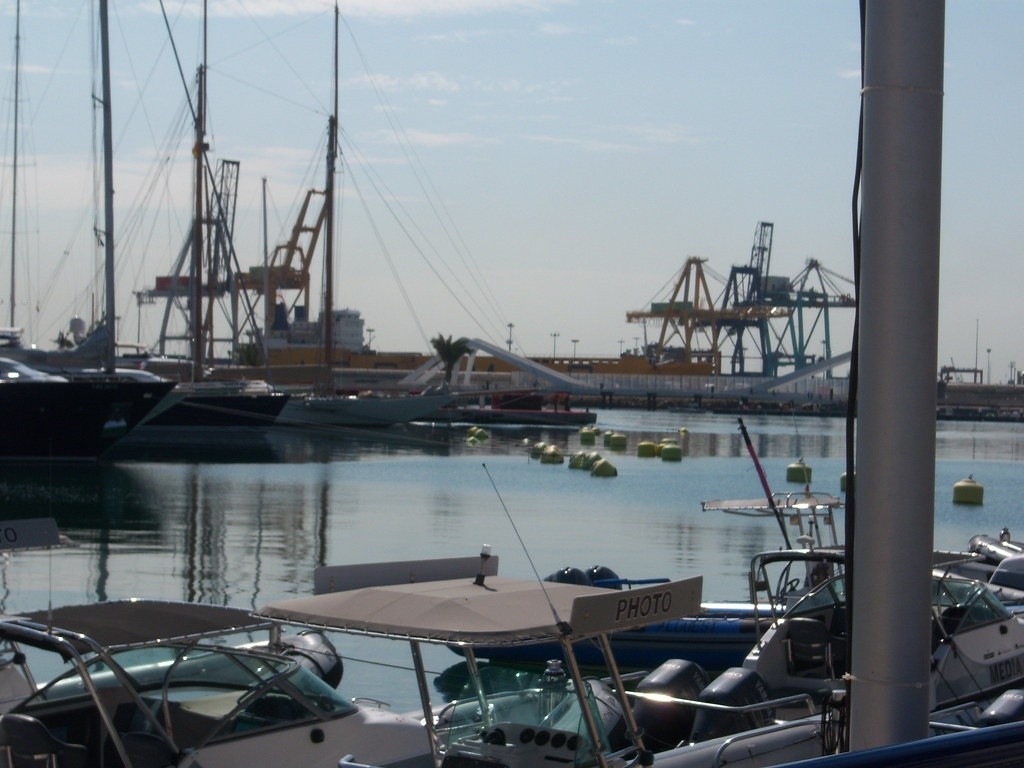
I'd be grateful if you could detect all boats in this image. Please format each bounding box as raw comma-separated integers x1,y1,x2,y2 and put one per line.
0,415,1024,768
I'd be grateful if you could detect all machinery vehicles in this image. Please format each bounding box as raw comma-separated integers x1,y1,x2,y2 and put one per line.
618,221,855,398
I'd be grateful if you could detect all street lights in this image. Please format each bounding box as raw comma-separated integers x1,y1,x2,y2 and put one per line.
550,332,559,359
571,339,579,365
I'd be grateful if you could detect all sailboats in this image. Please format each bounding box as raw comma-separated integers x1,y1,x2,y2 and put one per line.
1,1,601,429
